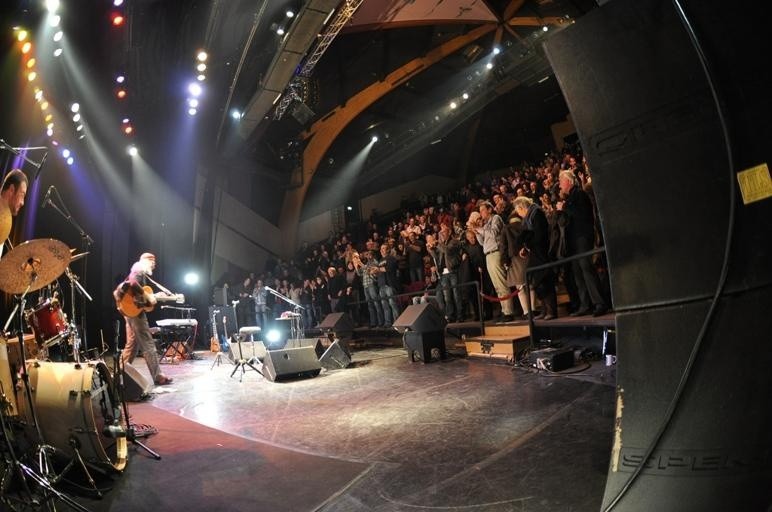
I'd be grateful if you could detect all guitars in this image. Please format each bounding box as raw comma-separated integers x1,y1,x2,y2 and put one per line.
114,283,185,318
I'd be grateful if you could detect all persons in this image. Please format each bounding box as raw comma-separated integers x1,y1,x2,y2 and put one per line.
0,167,30,255
112,252,174,386
222,140,615,332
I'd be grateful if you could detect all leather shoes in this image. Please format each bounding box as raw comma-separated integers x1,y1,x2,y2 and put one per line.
445,307,607,322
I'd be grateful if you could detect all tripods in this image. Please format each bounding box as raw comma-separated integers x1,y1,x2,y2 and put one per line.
227,299,265,383
207,348,232,370
0,295,161,512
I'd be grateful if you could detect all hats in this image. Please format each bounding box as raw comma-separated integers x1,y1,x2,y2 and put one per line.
140,252,155,261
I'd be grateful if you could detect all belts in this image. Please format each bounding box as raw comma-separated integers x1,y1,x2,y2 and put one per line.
484,250,497,254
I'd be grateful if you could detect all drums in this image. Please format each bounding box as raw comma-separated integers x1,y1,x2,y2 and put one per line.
16,357,129,473
28,298,71,349
6,336,49,367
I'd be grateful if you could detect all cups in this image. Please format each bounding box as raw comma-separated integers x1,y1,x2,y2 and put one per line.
605,355,616,366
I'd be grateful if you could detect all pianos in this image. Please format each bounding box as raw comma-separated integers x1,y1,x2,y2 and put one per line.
156,319,198,327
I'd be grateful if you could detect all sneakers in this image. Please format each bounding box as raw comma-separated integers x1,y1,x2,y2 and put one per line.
154,377,173,385
367,322,392,327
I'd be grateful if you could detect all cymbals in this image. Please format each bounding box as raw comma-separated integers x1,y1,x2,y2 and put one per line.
0,237,72,295
0,196,15,241
70,252,89,263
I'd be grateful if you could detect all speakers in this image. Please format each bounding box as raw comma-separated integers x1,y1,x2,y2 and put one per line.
264,120,301,146
260,346,322,381
282,336,324,355
320,338,353,369
318,310,353,332
214,287,228,305
393,302,448,333
227,336,269,363
209,305,238,343
